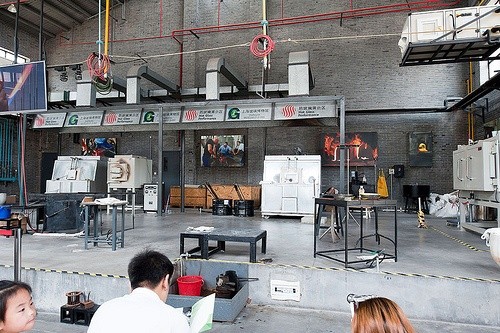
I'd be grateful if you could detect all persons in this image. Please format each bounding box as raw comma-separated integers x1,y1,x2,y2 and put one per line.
87,250,195,333
351,297,416,333
0,280,36,333
201,136,245,166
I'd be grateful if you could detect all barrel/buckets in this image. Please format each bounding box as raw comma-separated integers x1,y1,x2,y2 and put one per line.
0,193,7,204
0,205,12,220
177,276,204,296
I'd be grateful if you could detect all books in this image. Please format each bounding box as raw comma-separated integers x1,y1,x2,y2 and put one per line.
175,293,215,333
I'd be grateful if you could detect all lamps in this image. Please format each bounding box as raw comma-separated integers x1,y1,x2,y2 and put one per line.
7,4,17,13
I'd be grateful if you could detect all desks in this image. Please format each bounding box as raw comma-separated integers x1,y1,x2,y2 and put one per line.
314,198,398,268
82,201,129,251
180,227,267,263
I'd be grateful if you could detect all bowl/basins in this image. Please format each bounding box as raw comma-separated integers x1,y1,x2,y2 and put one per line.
66,291,82,305
360,193,380,200
